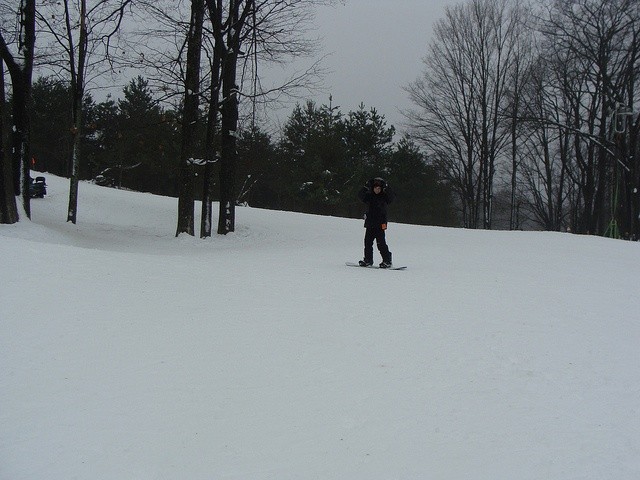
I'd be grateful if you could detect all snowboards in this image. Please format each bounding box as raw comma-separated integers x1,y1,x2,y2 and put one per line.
345,261,407,271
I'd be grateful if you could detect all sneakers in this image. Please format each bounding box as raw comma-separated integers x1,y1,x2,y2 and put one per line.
379,261,392,268
359,258,373,267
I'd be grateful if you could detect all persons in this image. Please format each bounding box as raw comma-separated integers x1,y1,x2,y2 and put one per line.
358,176,395,269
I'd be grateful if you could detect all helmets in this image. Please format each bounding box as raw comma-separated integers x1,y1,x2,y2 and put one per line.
370,177,385,187
